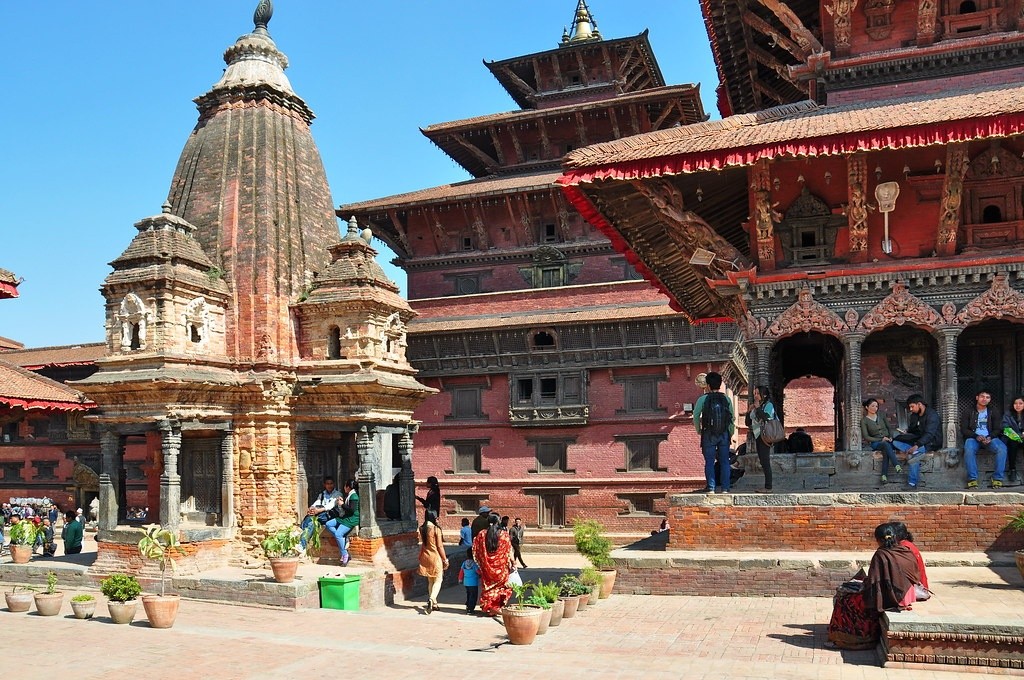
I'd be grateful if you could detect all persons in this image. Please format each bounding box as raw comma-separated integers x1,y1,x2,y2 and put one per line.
693,371,1024,650
303,476,529,617
0,502,147,558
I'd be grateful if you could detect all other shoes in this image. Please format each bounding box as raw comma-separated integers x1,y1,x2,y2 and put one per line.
722,490,728,494
991,476,1002,489
706,490,714,494
1007,469,1016,482
523,565,527,568
907,452,925,464
432,606,440,610
755,488,773,493
467,610,474,614
427,599,432,614
881,475,887,483
968,481,978,490
824,641,839,649
895,465,902,473
901,484,916,490
340,554,350,567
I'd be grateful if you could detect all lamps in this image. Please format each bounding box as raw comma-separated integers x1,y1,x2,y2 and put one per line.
875,182,900,253
690,248,740,270
3,433,13,443
683,403,694,414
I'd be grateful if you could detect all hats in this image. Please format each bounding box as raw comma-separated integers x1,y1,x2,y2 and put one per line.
479,506,491,513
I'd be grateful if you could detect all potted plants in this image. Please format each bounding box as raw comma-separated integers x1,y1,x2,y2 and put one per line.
34,571,64,616
138,523,186,628
1000,510,1024,579
260,515,320,584
9,519,45,564
70,594,97,618
502,566,604,645
100,574,143,625
571,515,616,599
5,586,39,613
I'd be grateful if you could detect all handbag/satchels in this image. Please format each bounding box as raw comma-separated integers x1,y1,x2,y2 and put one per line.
760,419,785,447
508,571,522,586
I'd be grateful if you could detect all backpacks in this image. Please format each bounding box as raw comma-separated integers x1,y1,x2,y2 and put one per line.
703,393,733,436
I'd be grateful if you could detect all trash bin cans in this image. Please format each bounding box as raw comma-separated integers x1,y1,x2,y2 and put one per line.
319,575,360,610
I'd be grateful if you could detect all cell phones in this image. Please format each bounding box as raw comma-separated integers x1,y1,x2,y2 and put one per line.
896,428,903,432
